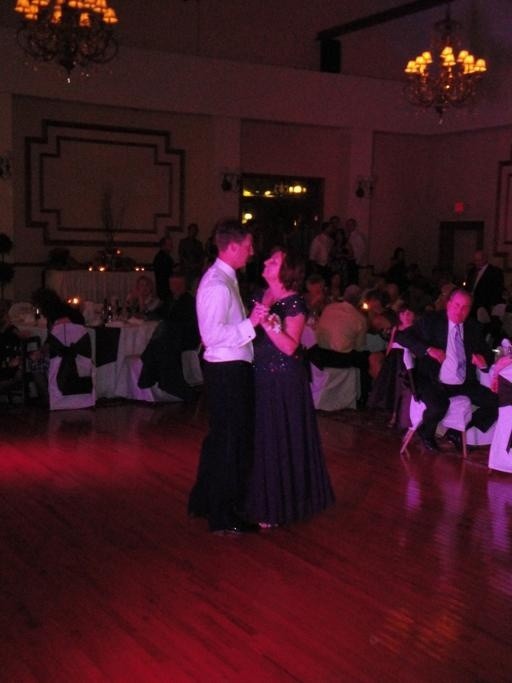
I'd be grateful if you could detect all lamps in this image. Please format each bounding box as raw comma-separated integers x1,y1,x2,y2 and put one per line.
400,0,489,124
12,0,119,83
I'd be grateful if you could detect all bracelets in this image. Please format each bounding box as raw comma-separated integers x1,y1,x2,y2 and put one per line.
261,314,284,333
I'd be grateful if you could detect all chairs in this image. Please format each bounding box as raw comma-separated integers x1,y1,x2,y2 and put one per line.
299,297,511,477
1,263,206,412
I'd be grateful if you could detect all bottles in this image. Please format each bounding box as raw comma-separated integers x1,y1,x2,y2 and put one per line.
103,298,109,322
34,306,41,325
106,303,113,321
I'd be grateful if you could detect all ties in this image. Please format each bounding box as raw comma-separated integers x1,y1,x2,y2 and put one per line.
454,323,467,383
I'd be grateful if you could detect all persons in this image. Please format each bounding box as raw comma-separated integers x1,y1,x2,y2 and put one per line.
2,222,222,404
187,220,265,532
249,244,336,526
302,215,512,453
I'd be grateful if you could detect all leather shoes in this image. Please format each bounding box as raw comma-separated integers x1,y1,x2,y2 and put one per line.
416,423,440,454
445,428,462,450
207,511,260,535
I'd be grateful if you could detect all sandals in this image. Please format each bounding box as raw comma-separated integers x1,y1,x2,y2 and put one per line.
386,416,398,429
